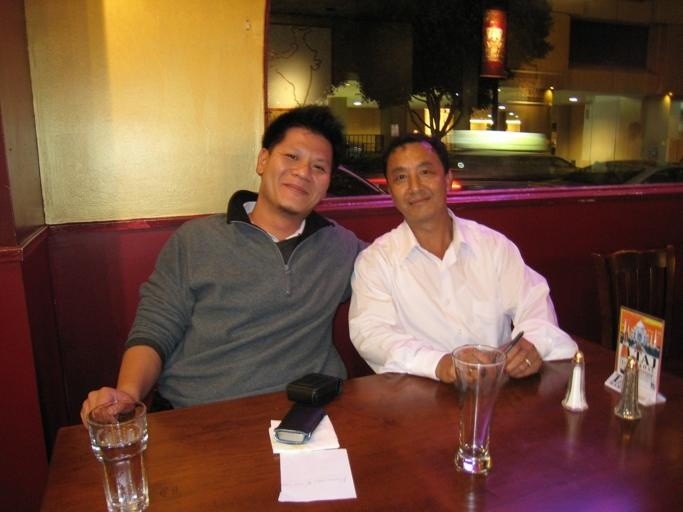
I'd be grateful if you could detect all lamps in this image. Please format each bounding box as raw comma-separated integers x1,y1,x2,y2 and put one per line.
480,0,509,79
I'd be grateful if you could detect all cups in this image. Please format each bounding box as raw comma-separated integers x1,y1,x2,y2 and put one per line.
451,343,506,477
83,400,150,512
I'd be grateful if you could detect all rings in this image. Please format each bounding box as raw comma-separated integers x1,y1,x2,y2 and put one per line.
525,358,532,367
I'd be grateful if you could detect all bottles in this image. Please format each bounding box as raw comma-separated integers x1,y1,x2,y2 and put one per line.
563,412,583,461
614,358,641,421
562,351,589,412
618,422,636,469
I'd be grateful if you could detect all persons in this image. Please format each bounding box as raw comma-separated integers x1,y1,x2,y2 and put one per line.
349,135,577,387
81,104,369,431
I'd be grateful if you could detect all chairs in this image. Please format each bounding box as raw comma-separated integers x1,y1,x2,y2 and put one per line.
590,241,683,373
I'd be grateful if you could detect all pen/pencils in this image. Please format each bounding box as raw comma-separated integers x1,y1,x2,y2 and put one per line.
504,331,523,353
